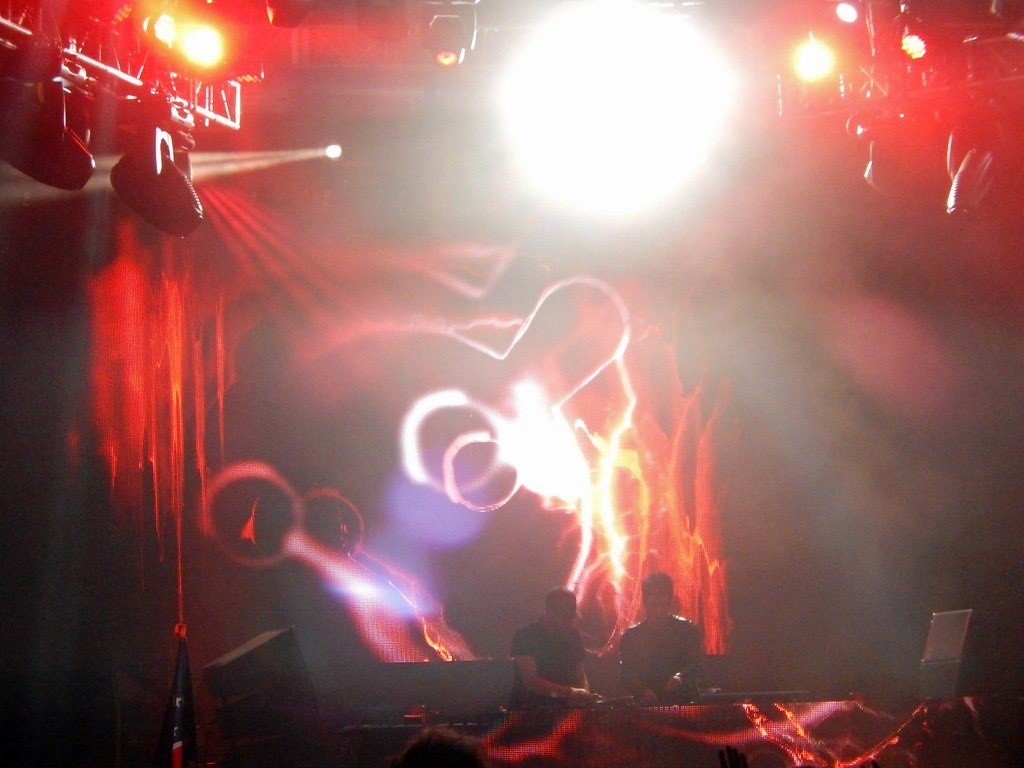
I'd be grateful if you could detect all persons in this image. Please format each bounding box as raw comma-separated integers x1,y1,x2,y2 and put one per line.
618,572,701,701
397,729,488,767
719,745,747,768
509,587,602,708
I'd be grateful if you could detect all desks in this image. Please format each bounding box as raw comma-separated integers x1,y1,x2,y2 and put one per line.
337,693,1011,768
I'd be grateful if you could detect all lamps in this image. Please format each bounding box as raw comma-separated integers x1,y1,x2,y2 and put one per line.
0,0,242,239
776,1,1024,224
